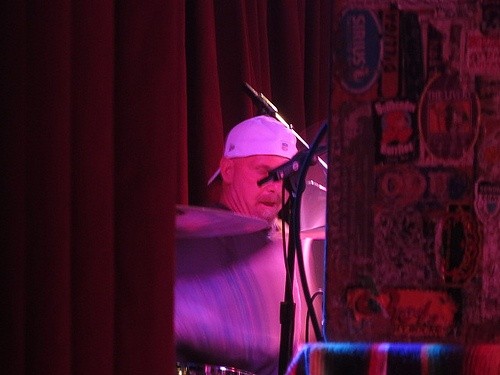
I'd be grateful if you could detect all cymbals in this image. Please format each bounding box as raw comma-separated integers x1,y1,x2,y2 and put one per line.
174,202,268,240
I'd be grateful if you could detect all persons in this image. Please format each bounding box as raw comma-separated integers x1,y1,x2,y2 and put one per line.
178,116,324,375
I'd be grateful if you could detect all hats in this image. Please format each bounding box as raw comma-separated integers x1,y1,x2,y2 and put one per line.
209,115,299,183
